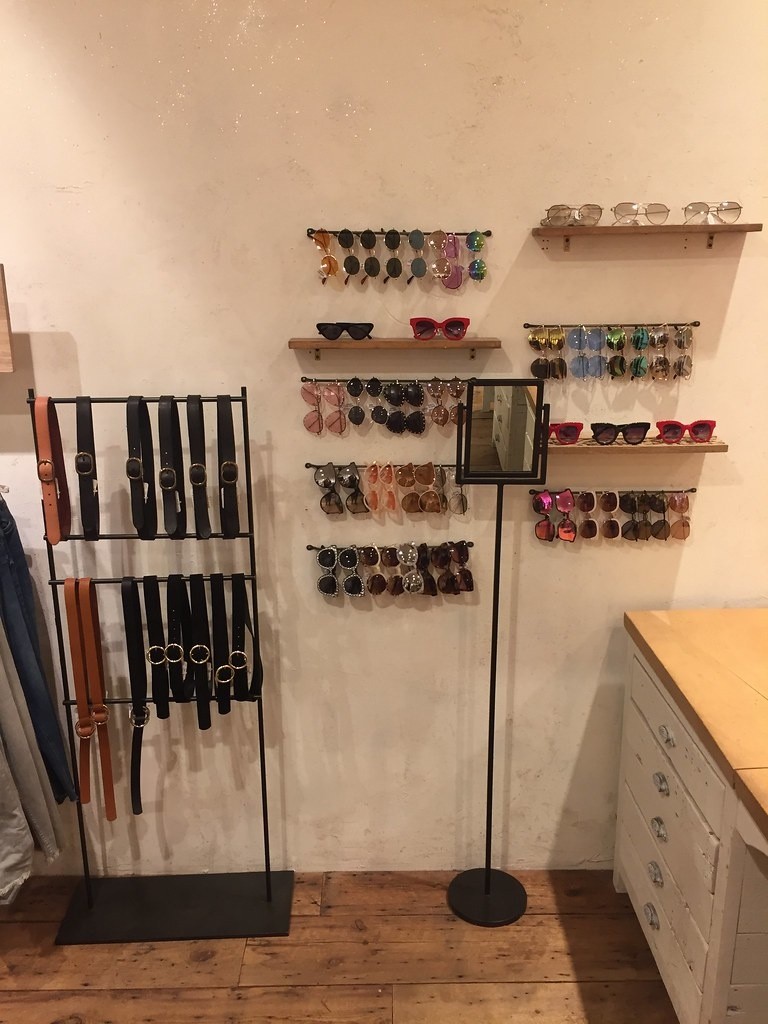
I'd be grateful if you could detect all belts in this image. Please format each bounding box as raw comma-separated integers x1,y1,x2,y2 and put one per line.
63,578,118,821
142,573,263,731
33,396,240,545
121,576,150,816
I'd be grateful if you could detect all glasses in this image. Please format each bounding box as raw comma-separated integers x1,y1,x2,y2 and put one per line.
610,202,671,225
528,325,693,381
682,201,742,225
313,229,485,291
313,461,468,516
316,322,374,341
656,420,716,444
316,540,476,598
591,422,651,445
302,375,466,436
533,489,690,543
543,204,604,227
548,423,583,445
410,318,469,340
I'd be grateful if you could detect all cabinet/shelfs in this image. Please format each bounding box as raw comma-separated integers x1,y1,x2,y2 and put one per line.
612,606,767,1024
